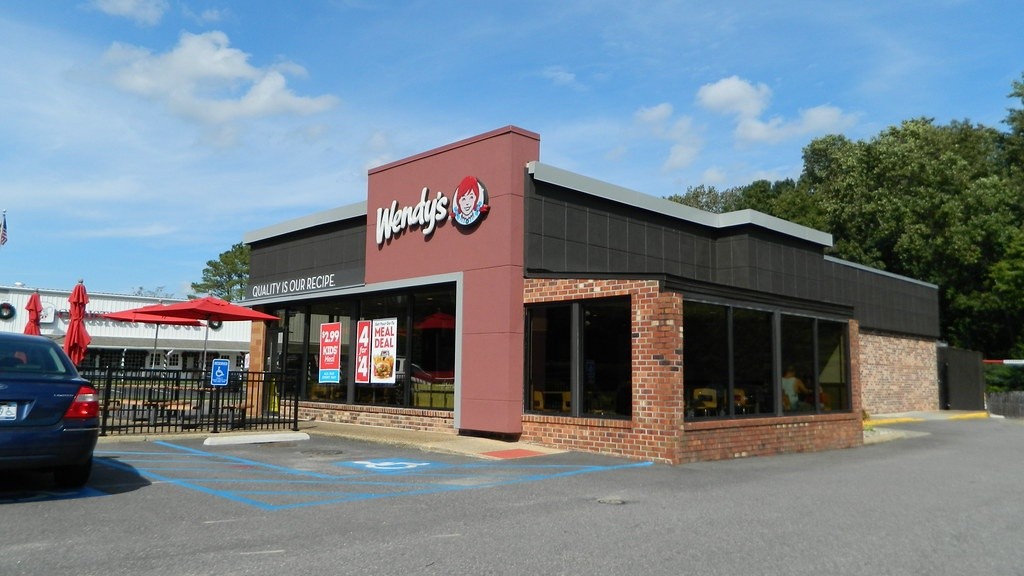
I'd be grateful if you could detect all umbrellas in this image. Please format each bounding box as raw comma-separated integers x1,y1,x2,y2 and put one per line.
64,279,93,367
133,293,282,387
24,288,44,337
95,299,207,368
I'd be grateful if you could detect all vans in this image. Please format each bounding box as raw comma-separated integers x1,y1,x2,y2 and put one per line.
396,358,406,385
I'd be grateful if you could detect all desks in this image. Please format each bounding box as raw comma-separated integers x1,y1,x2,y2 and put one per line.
138,385,218,427
94,385,106,435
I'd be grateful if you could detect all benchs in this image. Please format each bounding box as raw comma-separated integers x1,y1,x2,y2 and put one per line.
99,399,255,412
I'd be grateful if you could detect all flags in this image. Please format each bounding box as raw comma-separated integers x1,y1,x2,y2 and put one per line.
0,215,7,245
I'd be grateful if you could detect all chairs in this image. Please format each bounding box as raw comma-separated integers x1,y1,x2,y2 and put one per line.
533,388,832,417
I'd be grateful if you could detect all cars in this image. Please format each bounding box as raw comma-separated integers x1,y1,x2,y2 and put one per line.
0,332,101,491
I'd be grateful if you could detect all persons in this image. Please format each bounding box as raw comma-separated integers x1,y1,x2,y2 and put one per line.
780,365,813,411
